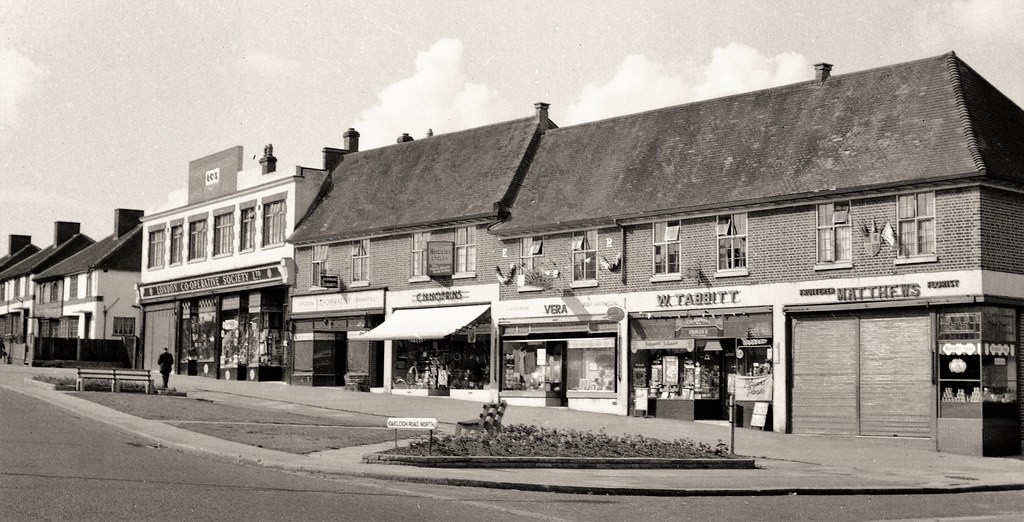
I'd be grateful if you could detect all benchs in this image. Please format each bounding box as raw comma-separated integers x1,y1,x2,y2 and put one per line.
76,367,151,394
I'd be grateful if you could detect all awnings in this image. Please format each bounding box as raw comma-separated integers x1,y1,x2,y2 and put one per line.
346,302,492,345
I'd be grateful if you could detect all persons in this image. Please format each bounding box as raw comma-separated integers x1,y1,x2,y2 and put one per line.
157,347,173,389
0,333,6,360
